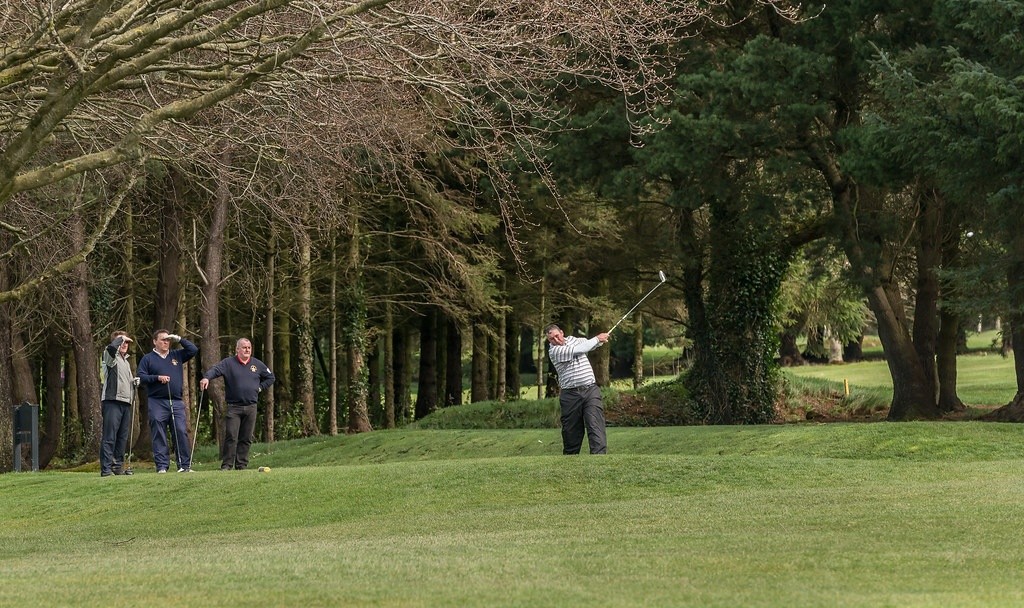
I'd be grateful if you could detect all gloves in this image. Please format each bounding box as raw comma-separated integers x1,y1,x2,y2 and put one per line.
133,377,140,385
161,334,181,343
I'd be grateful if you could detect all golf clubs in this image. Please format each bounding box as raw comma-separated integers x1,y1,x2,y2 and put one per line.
125,381,137,475
608,270,667,334
186,384,204,473
168,381,184,473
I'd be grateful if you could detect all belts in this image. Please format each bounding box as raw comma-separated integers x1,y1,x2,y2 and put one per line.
561,383,597,392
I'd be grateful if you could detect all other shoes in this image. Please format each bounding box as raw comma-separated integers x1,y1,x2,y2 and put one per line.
221,464,231,470
239,465,247,469
158,469,167,473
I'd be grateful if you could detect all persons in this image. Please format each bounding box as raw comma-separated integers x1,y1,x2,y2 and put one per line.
137,329,199,473
200,338,275,470
545,323,610,455
100,330,141,477
